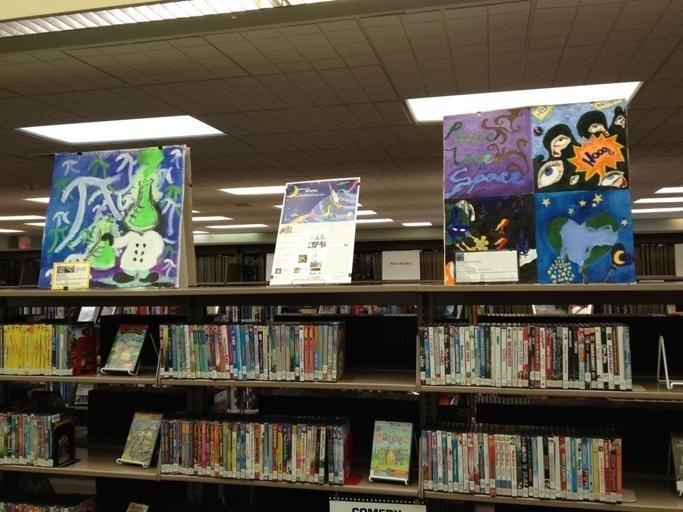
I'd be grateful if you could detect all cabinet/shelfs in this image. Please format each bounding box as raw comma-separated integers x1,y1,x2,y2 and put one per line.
0,274,683,512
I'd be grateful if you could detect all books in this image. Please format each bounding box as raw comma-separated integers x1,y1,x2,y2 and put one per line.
370,421,413,478
1,382,355,485
1,240,682,287
671,432,683,491
421,396,622,505
0,304,683,390
